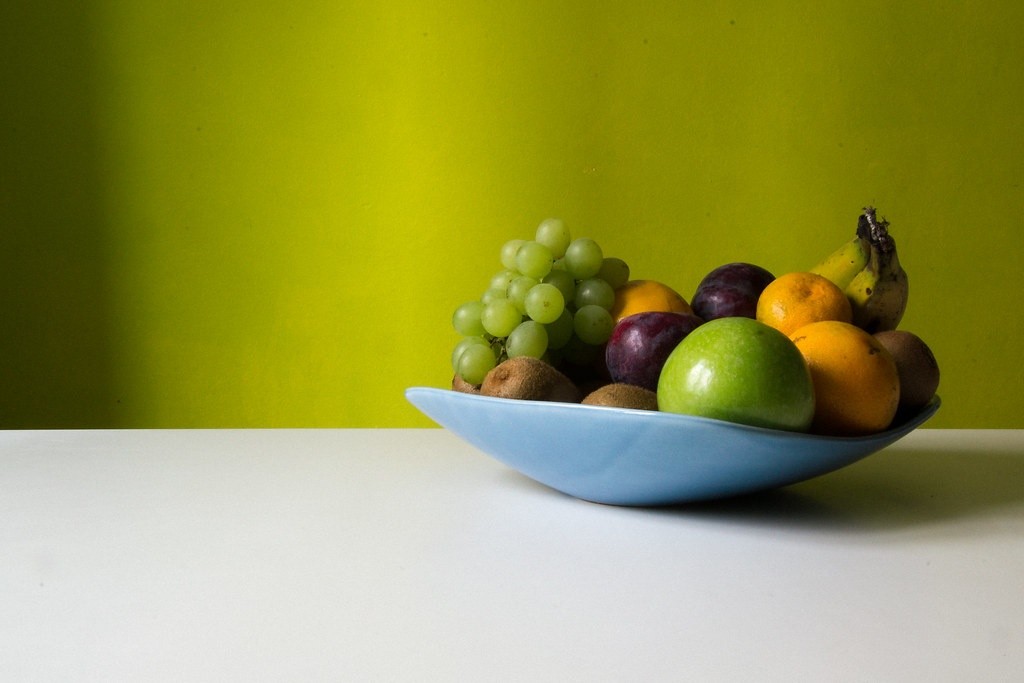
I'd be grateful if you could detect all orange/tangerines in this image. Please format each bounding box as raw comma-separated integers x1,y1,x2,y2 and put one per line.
757,272,853,337
615,279,694,321
787,321,900,435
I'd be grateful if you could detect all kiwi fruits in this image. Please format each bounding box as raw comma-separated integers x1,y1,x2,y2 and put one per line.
479,357,575,403
581,385,657,412
452,373,479,394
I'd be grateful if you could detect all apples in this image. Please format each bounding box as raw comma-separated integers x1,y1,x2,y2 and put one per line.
606,312,697,389
690,264,777,319
658,317,816,433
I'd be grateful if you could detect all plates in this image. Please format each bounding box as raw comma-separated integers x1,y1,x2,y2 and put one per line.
401,384,945,508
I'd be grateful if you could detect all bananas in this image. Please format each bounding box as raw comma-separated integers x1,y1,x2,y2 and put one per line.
807,205,910,332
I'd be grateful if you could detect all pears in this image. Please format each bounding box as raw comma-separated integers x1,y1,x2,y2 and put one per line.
871,330,941,407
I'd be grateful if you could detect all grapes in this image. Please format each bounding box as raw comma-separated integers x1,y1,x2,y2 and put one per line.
450,218,629,383
